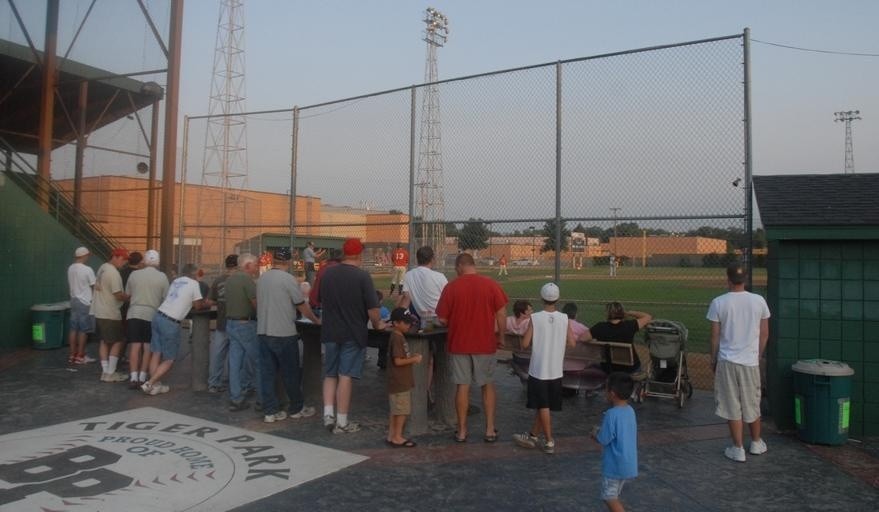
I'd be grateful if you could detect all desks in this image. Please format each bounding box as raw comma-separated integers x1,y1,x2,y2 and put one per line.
184,304,217,392
293,317,462,438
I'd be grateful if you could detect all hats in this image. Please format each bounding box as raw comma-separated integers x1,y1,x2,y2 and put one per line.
540,282,560,302
273,247,291,261
75,247,93,258
112,247,132,260
390,307,417,322
726,261,747,285
343,239,362,256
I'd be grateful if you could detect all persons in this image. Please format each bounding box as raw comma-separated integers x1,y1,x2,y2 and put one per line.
706,265,772,461
588,370,640,512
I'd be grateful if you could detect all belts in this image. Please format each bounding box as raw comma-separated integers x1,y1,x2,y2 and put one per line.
158,310,181,324
226,316,257,321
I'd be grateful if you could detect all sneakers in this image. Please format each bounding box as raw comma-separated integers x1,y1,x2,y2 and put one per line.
512,431,538,449
255,401,264,417
67,353,97,365
127,379,170,396
288,406,317,419
331,420,363,434
323,415,337,427
541,435,555,454
209,385,226,394
724,446,746,462
99,371,129,383
228,399,250,411
749,437,768,455
263,411,287,423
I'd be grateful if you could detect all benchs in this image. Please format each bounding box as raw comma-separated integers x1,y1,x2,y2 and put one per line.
486,330,644,410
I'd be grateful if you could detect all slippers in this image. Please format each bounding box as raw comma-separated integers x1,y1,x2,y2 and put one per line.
384,439,392,445
485,428,499,442
454,429,469,442
391,439,417,448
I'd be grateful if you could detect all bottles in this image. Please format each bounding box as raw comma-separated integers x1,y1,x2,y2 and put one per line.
420,309,432,331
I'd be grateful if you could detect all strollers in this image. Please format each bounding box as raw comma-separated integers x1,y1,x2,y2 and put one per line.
629,318,695,410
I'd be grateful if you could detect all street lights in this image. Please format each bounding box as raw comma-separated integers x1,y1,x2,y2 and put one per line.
412,3,452,256
528,225,537,263
833,109,868,174
608,206,622,256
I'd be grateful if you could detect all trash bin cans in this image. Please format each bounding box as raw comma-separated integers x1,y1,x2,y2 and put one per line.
29,301,78,350
791,359,855,446
